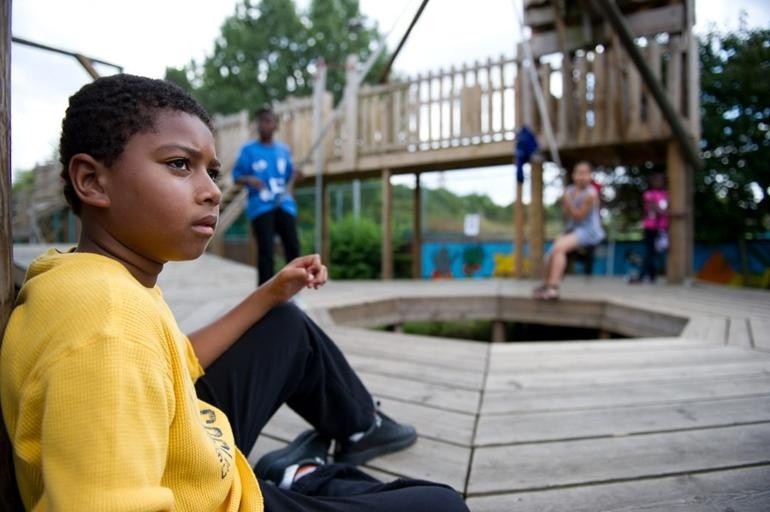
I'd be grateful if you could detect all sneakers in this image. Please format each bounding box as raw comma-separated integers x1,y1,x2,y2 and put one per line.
254,429,331,490
334,408,417,467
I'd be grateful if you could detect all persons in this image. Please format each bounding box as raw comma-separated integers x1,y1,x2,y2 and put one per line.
623,170,670,283
531,159,607,299
0,72,471,512
229,106,302,288
